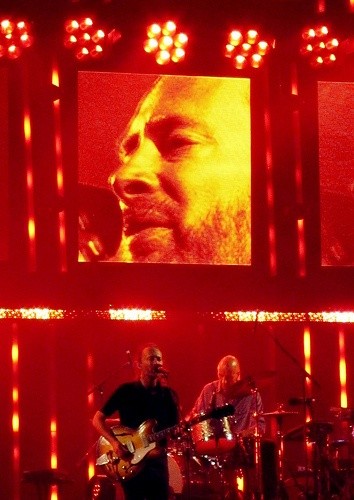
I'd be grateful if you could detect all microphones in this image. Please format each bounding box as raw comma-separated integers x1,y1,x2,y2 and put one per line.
289,398,312,406
154,365,169,374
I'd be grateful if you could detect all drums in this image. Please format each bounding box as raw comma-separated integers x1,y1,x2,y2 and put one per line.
167,453,221,500
192,409,235,452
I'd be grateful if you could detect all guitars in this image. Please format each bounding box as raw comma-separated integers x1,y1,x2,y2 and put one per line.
98,406,236,483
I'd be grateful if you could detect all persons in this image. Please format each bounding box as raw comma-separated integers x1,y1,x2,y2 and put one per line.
93,345,186,500
187,355,266,469
108,72,251,266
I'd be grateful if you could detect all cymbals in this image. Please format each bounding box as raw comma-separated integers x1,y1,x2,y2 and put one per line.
264,409,300,420
